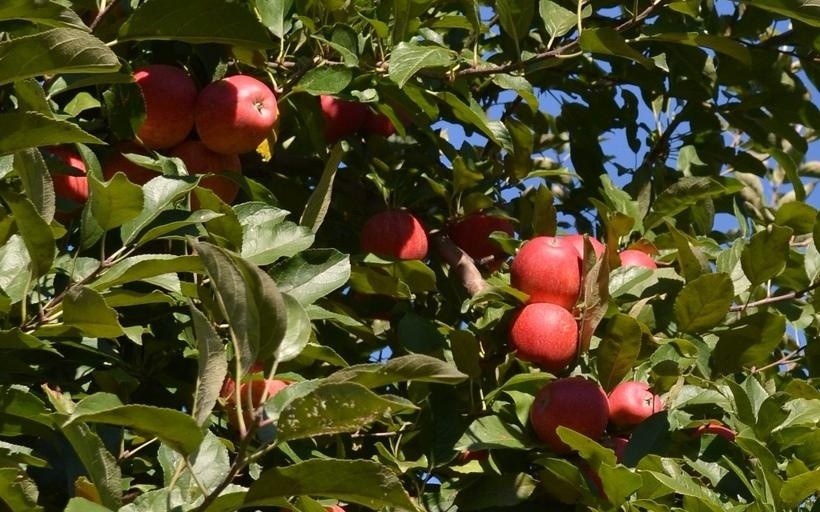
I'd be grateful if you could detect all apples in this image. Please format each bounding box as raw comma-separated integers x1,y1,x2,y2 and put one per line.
46,65,663,512
